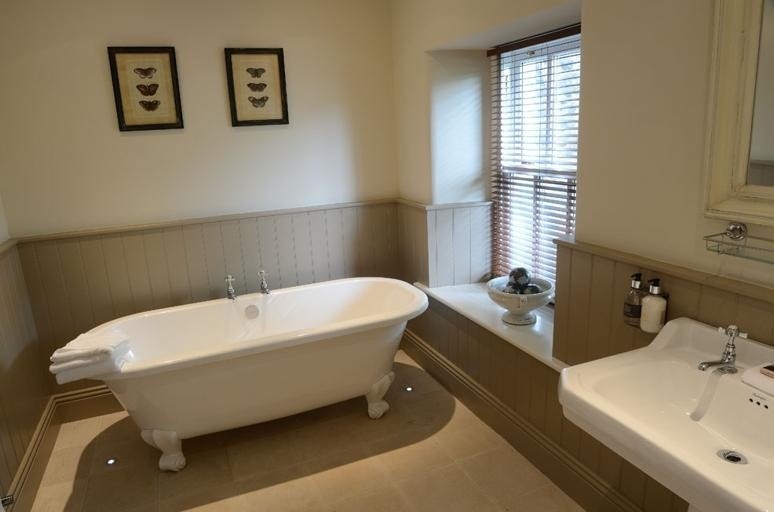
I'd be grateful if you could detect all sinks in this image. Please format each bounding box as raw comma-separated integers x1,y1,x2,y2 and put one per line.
556,315,773,512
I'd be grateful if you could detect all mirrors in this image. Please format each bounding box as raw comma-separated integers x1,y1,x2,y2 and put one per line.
705,1,774,230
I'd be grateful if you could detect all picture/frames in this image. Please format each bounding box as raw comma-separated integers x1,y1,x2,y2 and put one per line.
107,44,294,132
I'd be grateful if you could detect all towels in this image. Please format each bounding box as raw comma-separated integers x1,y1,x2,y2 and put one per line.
49,325,133,386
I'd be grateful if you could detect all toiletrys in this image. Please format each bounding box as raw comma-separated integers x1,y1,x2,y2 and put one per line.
638,278,667,333
622,272,644,327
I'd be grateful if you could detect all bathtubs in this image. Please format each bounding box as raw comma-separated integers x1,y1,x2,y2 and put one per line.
85,277,429,472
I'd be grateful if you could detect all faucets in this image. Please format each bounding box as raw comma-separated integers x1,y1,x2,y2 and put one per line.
698,324,740,372
223,274,236,301
257,270,270,296
